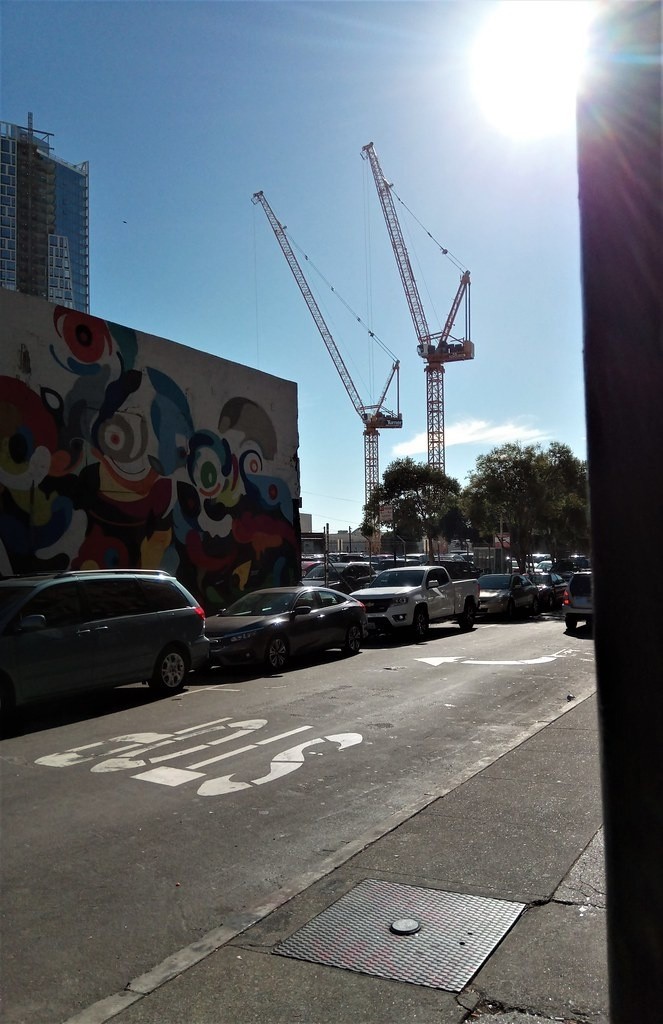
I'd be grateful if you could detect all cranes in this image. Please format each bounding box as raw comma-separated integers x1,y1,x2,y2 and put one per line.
249,189,404,552
363,141,475,552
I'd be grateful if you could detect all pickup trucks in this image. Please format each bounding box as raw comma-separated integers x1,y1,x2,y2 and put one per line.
349,565,481,639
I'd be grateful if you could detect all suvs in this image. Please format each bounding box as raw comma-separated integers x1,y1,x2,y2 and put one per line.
1,567,207,721
563,569,592,630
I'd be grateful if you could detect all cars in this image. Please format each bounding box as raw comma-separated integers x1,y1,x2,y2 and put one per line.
474,574,540,619
201,584,369,672
300,552,591,571
523,572,568,611
302,563,379,594
